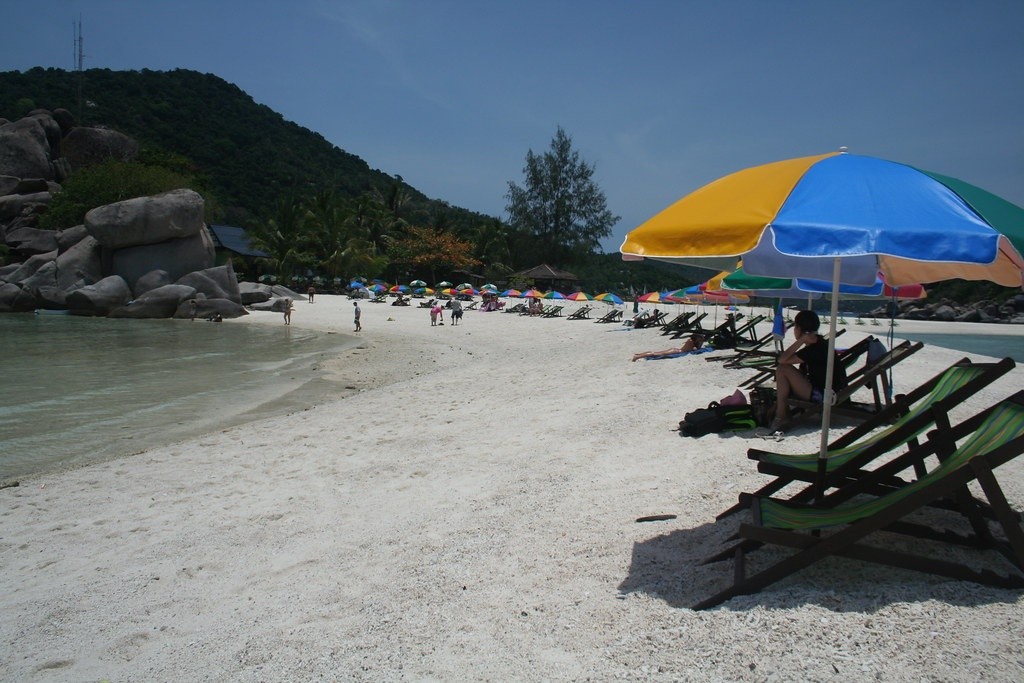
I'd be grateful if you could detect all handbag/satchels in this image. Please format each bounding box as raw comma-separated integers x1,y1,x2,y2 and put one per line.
750,385,774,427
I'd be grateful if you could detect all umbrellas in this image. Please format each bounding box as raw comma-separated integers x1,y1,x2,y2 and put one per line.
633,290,638,314
518,289,544,298
541,291,567,306
620,144,1024,538
344,280,498,302
772,298,786,353
697,258,927,309
593,293,624,314
567,292,594,308
500,289,522,308
639,281,752,330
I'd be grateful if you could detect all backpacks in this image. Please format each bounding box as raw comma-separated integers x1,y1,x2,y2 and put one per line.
680,401,727,436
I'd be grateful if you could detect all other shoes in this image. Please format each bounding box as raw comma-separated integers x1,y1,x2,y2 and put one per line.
757,422,792,438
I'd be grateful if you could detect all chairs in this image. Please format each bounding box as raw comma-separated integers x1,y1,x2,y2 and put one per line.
350,292,1024,611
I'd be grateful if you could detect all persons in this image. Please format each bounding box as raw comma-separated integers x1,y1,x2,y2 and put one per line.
396,294,402,305
419,297,462,326
284,299,293,325
632,334,704,362
761,311,848,434
635,309,659,322
353,302,362,332
308,286,315,303
530,299,544,317
